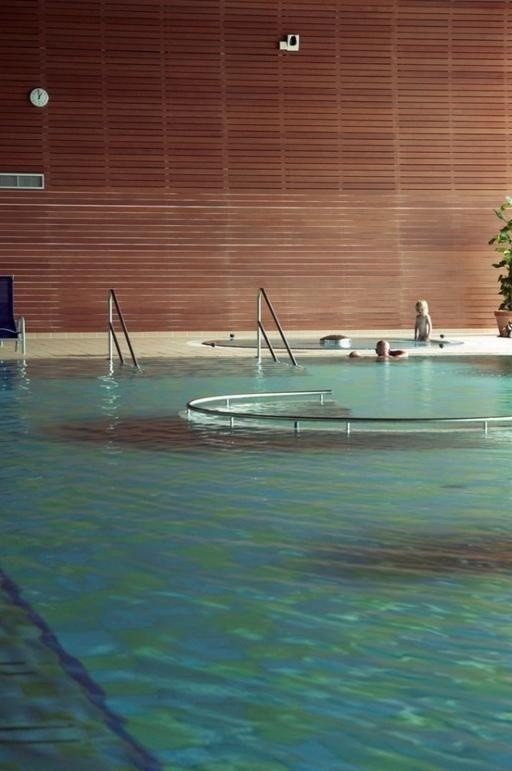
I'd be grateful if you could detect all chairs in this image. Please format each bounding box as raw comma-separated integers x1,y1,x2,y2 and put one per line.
1,273,26,359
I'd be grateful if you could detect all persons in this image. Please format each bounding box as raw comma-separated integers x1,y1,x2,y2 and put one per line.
348,338,408,362
413,298,432,344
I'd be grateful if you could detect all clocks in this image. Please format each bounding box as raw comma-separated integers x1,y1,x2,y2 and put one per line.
29,87,52,109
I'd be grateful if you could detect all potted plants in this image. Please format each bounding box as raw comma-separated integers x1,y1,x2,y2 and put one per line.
484,192,512,338
